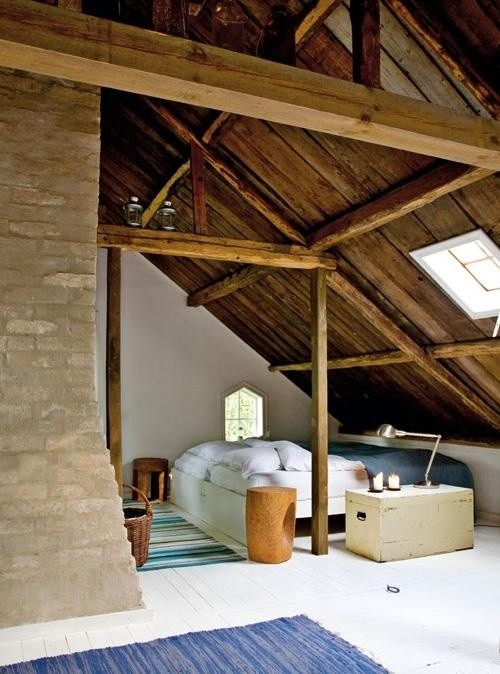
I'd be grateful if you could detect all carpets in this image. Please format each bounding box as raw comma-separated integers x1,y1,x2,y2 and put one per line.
0,615,393,674
123,491,248,572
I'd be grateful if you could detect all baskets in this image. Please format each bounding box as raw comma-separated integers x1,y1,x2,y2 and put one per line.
122,482,153,566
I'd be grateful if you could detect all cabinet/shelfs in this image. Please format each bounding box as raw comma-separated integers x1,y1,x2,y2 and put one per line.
345,483,474,563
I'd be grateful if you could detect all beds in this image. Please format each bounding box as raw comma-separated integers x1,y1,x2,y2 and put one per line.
170,442,475,548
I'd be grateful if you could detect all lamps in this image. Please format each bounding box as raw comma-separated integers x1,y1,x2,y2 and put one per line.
375,423,443,488
157,201,176,231
122,196,143,227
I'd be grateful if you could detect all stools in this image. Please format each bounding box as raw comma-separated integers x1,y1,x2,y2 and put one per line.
133,458,168,503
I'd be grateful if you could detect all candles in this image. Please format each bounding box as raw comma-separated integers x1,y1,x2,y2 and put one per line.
373,472,383,490
388,474,400,488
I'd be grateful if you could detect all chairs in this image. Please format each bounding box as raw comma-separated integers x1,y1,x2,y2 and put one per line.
246,486,297,564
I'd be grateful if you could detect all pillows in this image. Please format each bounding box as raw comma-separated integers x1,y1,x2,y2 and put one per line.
185,441,284,479
